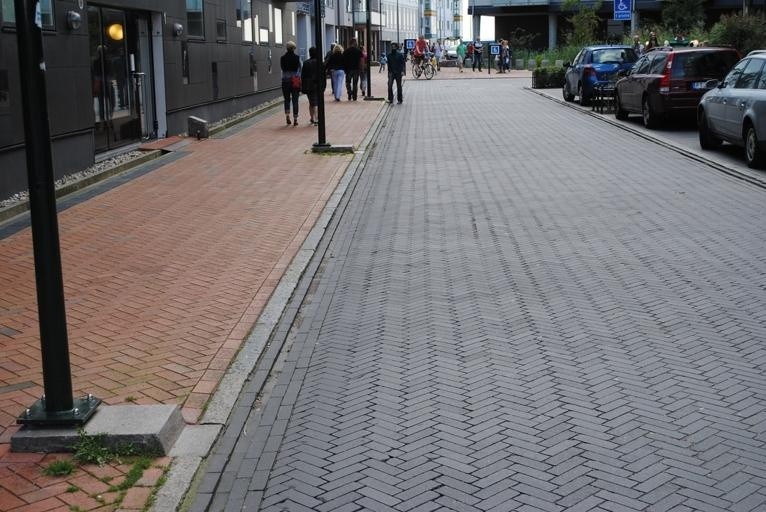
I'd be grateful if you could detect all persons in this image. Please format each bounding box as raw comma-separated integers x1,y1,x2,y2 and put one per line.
386,42,406,105
114,47,131,106
359,39,367,96
403,36,513,79
91,44,116,120
646,31,661,50
324,42,336,94
326,45,345,100
630,34,644,58
279,40,303,126
671,28,689,47
342,38,361,100
378,52,388,73
301,46,326,126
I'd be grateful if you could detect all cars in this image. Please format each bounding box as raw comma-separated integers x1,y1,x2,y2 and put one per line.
562,46,641,108
444,45,470,61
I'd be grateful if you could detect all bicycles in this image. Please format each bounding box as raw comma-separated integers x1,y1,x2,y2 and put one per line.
411,53,435,80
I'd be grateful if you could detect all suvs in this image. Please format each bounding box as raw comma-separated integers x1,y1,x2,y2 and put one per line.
697,49,766,169
612,45,744,129
